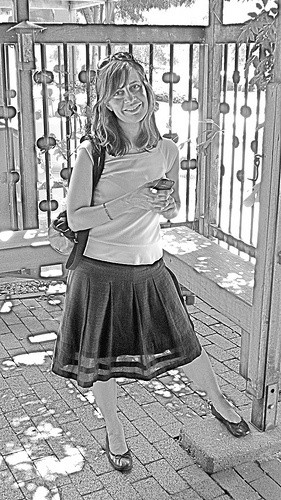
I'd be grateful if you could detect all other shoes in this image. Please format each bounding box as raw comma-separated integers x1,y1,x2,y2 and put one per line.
105,431,132,472
209,396,253,439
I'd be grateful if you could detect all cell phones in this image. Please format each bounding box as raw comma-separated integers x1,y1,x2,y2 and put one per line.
153,178,176,190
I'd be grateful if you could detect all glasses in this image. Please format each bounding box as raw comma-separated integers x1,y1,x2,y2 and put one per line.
94,49,133,73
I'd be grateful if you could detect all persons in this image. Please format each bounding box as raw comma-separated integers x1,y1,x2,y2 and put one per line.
48,51,250,474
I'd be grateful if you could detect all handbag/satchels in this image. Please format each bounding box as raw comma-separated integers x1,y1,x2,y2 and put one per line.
51,132,107,271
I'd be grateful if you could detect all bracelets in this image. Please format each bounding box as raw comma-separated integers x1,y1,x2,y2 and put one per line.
101,202,112,221
